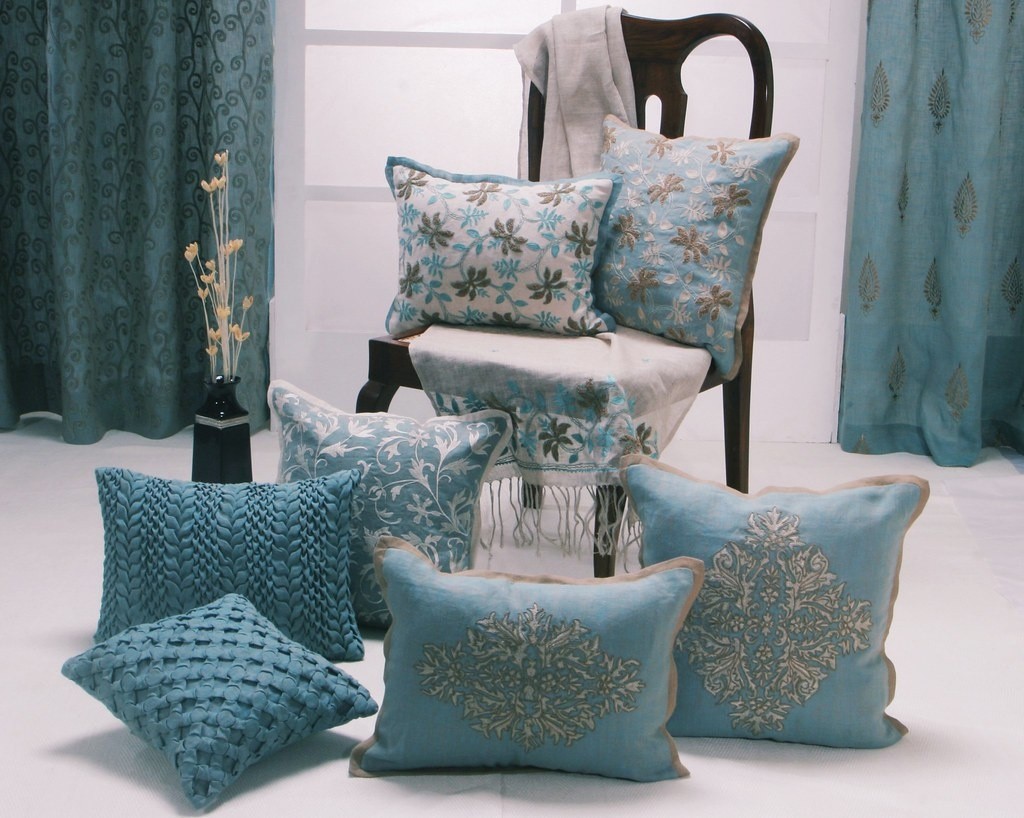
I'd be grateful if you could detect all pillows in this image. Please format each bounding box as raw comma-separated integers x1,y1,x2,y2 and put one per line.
266,381,513,631
617,452,931,750
92,466,367,664
62,595,378,811
385,156,617,340
349,535,706,784
592,113,800,385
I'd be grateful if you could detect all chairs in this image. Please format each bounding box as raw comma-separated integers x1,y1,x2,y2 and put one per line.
356,3,775,576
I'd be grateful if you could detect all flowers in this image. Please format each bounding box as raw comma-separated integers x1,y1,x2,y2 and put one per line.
185,150,254,384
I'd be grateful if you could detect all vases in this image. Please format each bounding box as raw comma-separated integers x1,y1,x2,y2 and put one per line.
191,376,253,483
